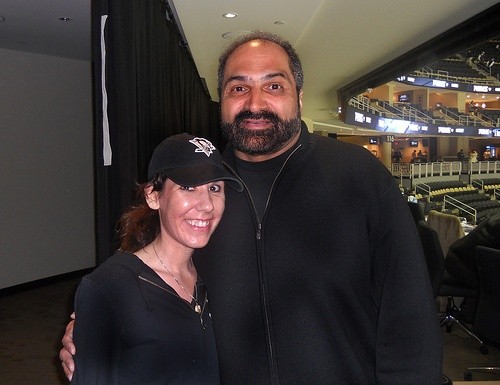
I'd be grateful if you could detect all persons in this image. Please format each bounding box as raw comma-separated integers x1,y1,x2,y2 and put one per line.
72,133,244,385
399,188,500,323
487,57,495,70
61,29,445,384
394,148,478,163
478,51,485,69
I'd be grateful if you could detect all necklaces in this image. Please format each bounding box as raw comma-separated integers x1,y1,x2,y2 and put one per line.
153,242,202,315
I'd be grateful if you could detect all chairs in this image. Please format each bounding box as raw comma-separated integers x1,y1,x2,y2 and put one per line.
406,178,500,257
418,209,500,355
348,95,500,127
408,59,500,86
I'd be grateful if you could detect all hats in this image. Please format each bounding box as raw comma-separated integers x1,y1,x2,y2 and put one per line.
147,132,244,192
